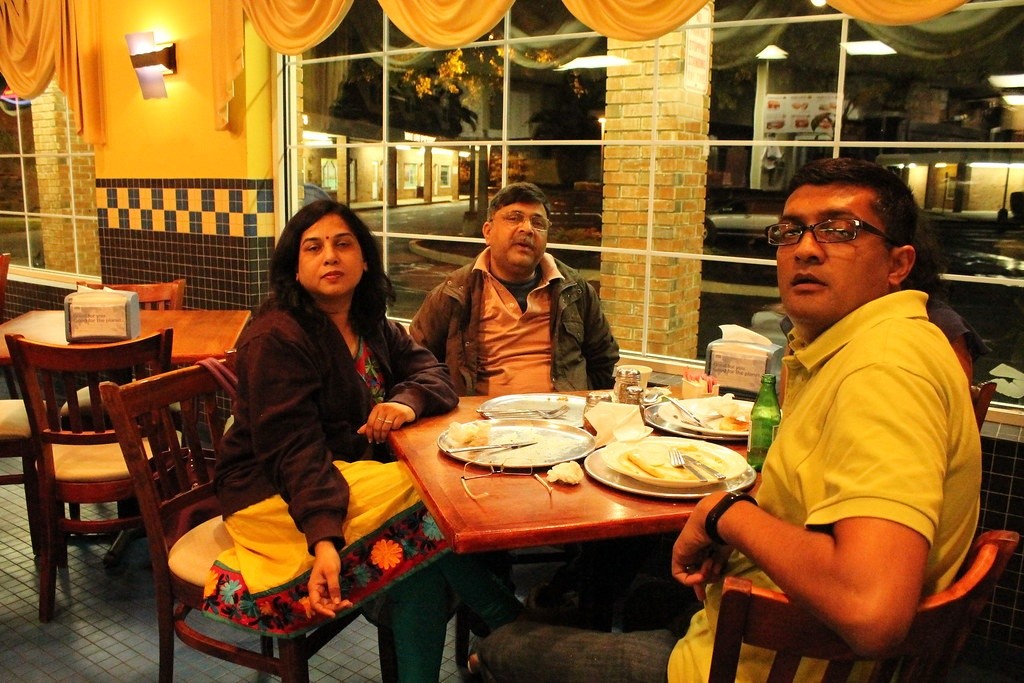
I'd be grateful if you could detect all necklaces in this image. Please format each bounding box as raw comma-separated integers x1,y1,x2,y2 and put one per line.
321,310,352,314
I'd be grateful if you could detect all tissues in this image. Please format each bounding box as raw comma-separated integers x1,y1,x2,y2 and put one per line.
746,310,794,347
704,323,784,402
62,284,140,344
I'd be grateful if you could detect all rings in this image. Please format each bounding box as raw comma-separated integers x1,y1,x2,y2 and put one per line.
385,420,392,423
378,418,384,421
686,566,695,570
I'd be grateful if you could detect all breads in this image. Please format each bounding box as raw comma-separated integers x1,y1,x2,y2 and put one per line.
718,412,751,432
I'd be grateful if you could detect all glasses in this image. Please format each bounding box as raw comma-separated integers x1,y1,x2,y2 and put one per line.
764,219,901,246
500,211,553,231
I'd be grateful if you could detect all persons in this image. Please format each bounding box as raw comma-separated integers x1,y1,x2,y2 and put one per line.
408,181,634,683
474,157,983,683
216,199,536,683
764,133,782,186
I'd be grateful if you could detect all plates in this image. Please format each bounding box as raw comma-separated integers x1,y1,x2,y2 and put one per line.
659,396,754,435
643,398,783,441
479,393,590,428
583,438,757,500
604,437,748,488
437,418,596,466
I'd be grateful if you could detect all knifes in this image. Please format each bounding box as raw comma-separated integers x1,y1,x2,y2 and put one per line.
681,455,726,480
666,397,713,428
446,441,538,454
476,410,551,413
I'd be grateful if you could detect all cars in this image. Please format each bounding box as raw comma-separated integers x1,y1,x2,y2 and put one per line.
705,212,782,260
938,249,1024,344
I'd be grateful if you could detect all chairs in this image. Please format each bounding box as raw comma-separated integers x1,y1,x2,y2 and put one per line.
0,251,363,683
708,530,1019,683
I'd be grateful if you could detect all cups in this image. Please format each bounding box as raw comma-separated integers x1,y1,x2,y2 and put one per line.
618,364,652,391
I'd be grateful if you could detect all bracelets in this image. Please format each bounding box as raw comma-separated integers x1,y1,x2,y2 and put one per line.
703,490,758,544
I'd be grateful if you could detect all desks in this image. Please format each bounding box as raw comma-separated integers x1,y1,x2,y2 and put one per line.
0,310,251,521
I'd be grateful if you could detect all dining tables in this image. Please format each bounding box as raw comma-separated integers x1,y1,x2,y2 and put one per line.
384,386,761,683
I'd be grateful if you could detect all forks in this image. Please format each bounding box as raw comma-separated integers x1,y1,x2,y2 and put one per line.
481,405,569,419
667,448,708,482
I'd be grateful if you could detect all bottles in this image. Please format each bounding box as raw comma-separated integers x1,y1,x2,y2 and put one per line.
613,368,640,405
626,386,644,407
583,392,612,435
747,374,781,471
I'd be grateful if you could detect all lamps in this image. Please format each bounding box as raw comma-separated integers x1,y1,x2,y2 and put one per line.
756,44,788,59
125,32,177,99
556,43,631,70
